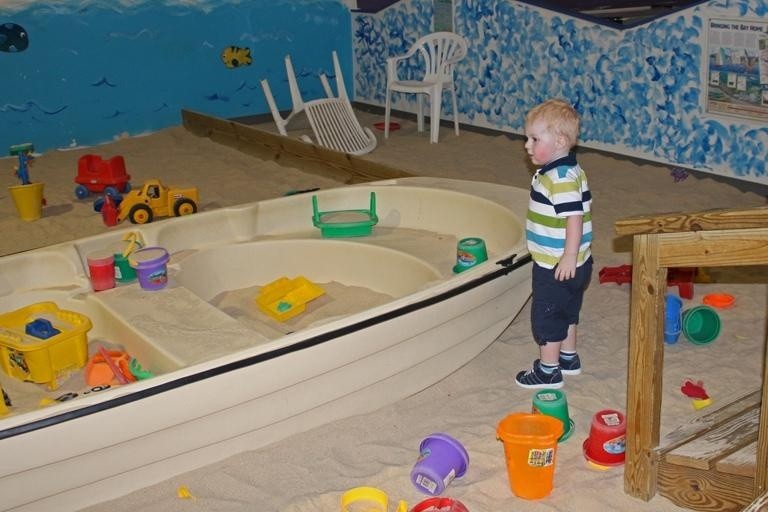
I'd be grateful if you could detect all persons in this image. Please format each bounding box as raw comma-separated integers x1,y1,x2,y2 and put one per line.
511,98,602,389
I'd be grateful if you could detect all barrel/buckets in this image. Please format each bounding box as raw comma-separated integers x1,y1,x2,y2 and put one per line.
453,237,488,274
111,231,171,292
7,181,44,223
533,388,576,443
409,434,469,496
582,409,627,470
681,304,720,345
495,414,564,500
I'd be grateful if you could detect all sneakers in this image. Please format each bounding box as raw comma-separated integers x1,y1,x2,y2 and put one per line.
515,353,580,388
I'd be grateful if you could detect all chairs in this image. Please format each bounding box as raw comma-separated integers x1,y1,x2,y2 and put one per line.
379,32,471,146
260,50,379,157
